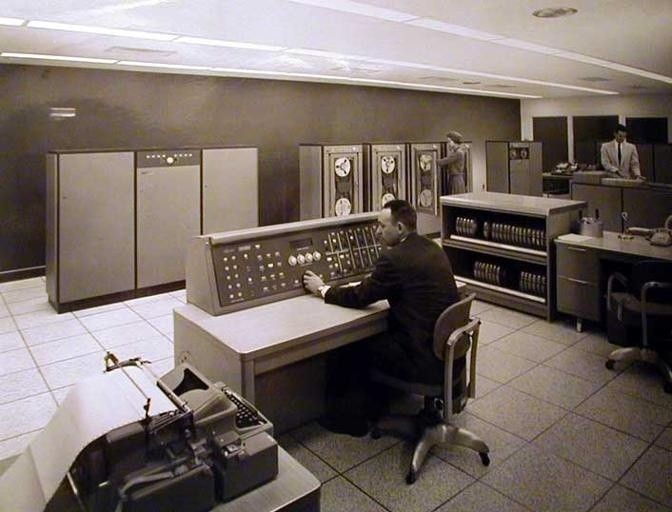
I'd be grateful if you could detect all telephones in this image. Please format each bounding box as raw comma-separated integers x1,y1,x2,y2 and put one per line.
645,228,672,246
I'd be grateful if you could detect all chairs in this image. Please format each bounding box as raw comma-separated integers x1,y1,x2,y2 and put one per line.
605,262,672,394
369,293,491,483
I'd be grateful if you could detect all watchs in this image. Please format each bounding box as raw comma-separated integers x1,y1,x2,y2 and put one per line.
317,283,328,298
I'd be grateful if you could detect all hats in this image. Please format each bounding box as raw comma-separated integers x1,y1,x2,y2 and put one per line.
449,131,463,144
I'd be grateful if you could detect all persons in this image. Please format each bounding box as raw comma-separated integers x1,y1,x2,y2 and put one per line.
437,130,466,194
600,124,644,179
303,199,460,438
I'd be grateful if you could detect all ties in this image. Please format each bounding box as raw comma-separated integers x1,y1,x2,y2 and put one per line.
618,143,621,162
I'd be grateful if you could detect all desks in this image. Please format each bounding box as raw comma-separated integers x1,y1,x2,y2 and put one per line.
173,277,470,411
1,444,322,512
550,226,672,334
570,178,672,233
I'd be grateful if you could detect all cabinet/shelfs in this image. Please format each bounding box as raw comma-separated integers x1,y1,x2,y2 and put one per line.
439,191,588,324
